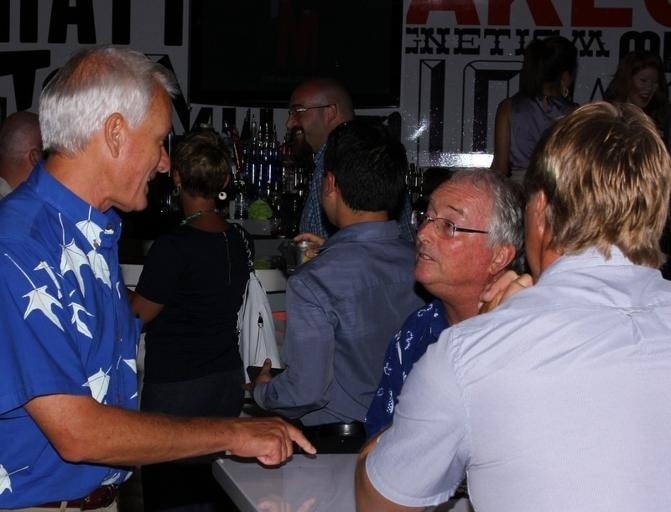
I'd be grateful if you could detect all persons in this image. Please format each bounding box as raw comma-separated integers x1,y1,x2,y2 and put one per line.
489,33,581,186
240,115,430,453
354,99,671,512
122,125,252,510
1,39,319,511
282,77,418,248
362,166,525,440
603,48,671,140
1,110,44,201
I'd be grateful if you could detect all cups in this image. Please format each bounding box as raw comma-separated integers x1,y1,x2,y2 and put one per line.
278,238,315,277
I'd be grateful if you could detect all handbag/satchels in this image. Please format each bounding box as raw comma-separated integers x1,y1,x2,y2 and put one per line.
238,270,282,399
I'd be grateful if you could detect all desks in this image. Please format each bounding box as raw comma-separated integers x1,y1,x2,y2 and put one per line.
210,452,358,512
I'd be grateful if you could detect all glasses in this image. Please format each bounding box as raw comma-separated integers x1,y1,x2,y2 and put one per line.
289,104,331,115
411,210,486,240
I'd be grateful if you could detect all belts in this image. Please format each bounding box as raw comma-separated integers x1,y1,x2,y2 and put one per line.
43,484,117,509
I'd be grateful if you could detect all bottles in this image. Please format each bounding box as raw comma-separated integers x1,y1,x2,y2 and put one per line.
225,117,311,236
404,163,425,226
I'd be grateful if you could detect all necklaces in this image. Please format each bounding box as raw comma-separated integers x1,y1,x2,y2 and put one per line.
180,207,221,225
